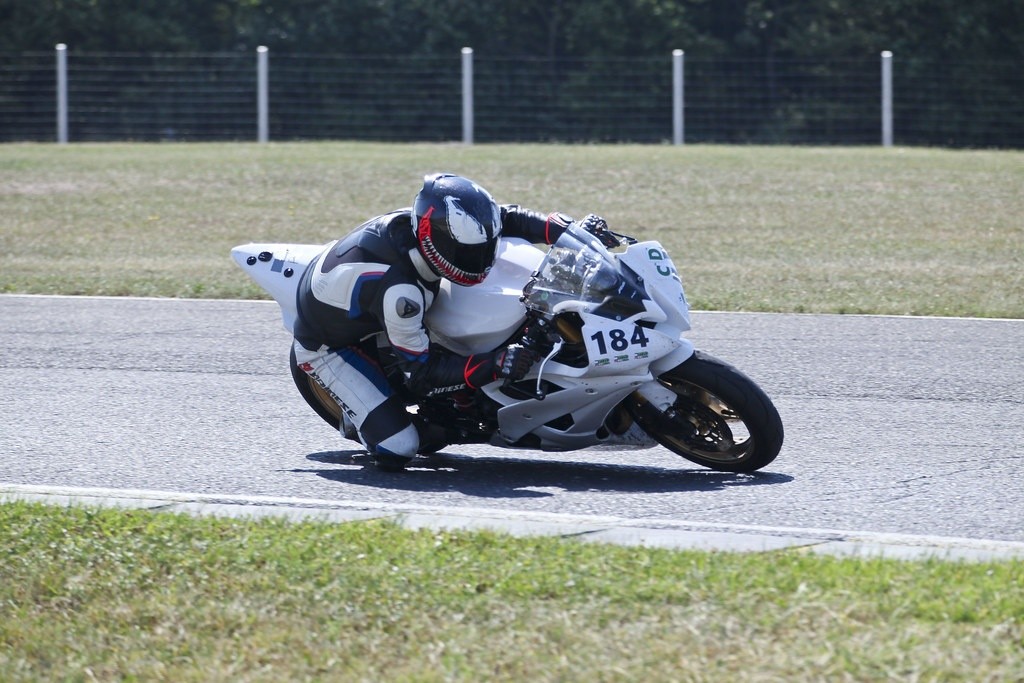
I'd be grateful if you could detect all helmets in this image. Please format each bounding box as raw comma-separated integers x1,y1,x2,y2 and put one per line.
410,174,502,287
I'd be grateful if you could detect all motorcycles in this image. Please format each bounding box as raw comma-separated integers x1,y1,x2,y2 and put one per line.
229,219,785,473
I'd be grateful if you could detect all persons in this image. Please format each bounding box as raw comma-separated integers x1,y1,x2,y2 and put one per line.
296,174,620,464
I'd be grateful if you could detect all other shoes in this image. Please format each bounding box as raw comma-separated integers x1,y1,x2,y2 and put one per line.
407,412,448,456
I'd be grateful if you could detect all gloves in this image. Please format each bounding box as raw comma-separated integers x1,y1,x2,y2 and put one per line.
463,343,541,390
548,212,620,249
